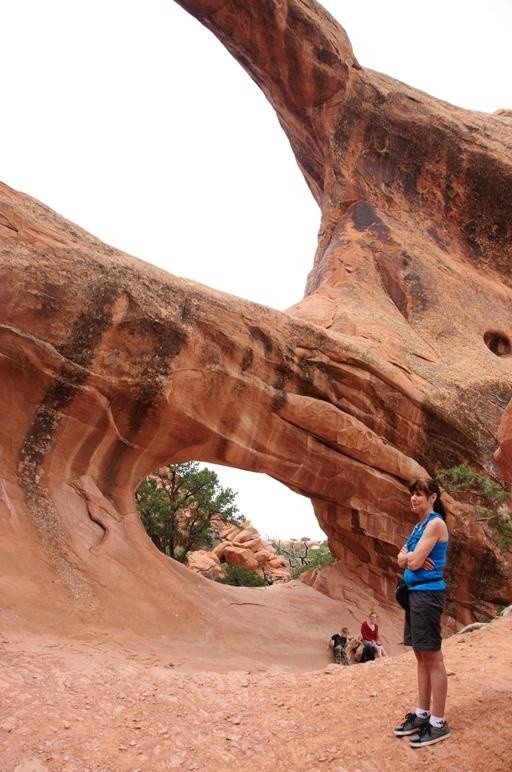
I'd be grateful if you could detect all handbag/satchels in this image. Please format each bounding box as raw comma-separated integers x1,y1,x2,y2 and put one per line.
394,576,445,610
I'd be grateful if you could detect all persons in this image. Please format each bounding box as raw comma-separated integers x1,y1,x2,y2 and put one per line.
328,627,352,665
361,612,388,659
390,476,453,749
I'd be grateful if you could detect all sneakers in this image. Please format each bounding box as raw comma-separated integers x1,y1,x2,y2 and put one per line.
408,714,452,748
393,713,431,737
